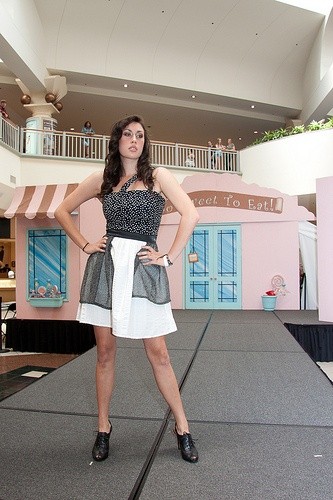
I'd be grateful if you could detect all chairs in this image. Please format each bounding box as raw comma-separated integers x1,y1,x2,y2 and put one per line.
1,303,16,343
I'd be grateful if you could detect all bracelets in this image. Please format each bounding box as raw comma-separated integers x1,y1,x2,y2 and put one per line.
163,255,172,268
82,242,90,251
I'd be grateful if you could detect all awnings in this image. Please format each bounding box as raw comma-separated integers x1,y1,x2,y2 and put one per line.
3,183,79,218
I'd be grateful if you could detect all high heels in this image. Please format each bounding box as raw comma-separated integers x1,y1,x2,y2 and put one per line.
175,422,198,463
92,420,112,462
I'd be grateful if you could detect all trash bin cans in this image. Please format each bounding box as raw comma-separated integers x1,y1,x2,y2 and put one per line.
262,295,276,312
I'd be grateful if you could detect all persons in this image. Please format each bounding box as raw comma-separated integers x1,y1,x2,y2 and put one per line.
0,246,5,268
81,120,95,157
185,137,235,171
53,116,200,462
0,99,10,119
